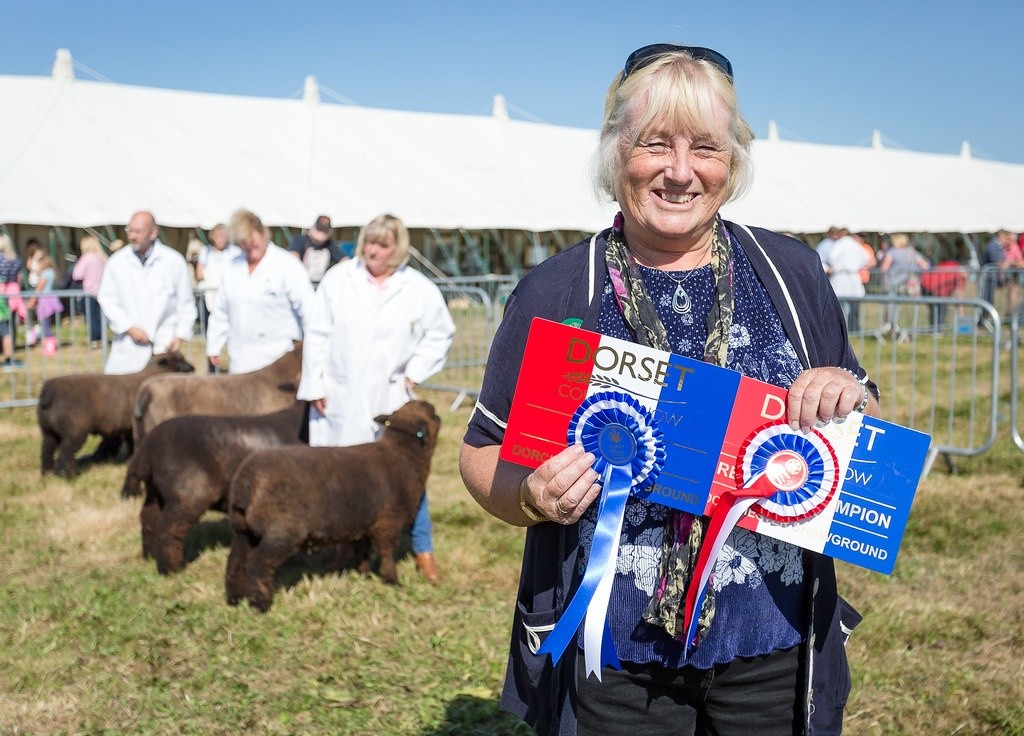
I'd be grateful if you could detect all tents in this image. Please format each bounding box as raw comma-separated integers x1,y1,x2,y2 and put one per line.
0,49,1023,236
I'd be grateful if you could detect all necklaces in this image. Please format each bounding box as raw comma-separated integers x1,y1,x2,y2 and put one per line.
624,231,707,315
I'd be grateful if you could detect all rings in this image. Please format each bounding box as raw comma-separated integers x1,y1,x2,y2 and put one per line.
556,498,571,514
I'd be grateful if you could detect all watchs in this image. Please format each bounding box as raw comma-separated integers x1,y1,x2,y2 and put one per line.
520,475,552,522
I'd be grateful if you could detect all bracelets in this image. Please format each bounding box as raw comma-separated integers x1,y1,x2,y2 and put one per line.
835,366,868,412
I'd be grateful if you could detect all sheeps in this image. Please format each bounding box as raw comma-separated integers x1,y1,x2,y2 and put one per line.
134,341,308,441
120,403,311,574
36,348,195,478
224,398,441,611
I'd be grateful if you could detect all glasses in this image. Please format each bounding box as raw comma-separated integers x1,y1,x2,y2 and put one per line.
619,44,734,86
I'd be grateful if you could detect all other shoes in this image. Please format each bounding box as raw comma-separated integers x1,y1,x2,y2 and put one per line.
3,359,24,370
414,551,439,581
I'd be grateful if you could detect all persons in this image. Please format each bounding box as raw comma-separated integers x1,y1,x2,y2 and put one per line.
0,212,1024,587
457,43,880,736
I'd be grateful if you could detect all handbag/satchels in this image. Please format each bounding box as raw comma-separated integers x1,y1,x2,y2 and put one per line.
905,274,920,296
